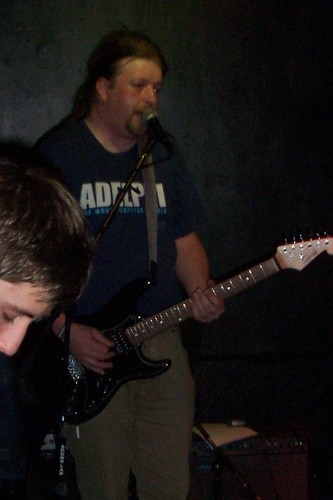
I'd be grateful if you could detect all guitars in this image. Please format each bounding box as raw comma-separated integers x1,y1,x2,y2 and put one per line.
48,231,333,426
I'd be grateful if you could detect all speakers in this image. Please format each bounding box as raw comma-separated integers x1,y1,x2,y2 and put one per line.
183,436,319,500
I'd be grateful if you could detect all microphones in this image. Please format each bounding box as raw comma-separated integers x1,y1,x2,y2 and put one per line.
141,108,175,159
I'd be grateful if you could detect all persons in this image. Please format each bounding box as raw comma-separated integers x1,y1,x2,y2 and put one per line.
28,28,226,499
0,157,97,357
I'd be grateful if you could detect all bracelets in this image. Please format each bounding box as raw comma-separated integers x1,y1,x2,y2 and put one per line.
57,322,66,338
